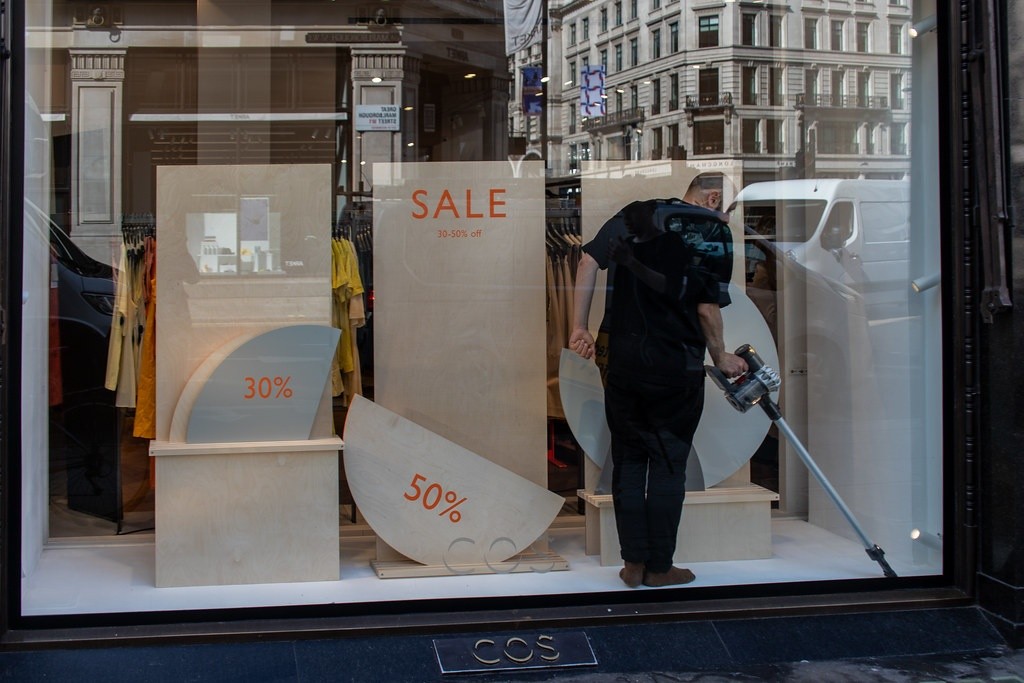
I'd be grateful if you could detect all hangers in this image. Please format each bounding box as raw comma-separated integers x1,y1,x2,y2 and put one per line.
121,221,156,259
332,217,373,260
546,206,582,255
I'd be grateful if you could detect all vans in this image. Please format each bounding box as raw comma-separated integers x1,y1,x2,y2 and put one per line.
725,177,910,320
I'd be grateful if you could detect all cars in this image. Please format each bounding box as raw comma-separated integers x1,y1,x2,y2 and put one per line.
19,194,117,437
639,198,874,440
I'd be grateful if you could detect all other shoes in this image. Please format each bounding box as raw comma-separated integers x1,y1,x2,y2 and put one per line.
643,563,696,586
619,560,644,588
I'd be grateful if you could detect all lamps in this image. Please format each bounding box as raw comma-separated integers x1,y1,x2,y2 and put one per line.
909,528,942,547
908,15,937,38
911,274,940,292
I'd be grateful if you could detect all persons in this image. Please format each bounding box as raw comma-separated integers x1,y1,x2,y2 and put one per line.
569,172,748,588
